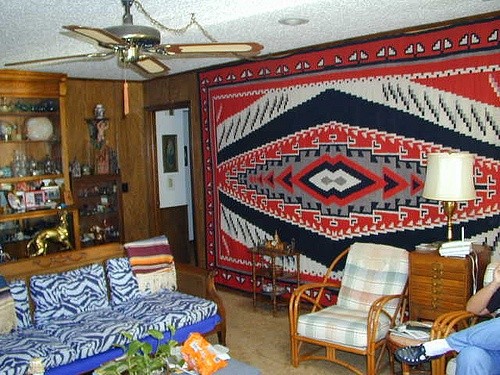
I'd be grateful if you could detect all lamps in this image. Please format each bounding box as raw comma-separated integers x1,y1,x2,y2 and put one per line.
98,0,161,64
422,154,478,243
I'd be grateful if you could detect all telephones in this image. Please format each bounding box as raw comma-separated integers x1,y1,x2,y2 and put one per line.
439,240,473,258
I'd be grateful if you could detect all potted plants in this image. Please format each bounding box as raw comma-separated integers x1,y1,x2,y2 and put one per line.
92,325,186,375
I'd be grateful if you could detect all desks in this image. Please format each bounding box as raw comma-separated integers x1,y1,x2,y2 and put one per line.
248,248,301,317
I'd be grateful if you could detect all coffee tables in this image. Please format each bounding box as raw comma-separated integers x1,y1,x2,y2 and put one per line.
93,345,261,375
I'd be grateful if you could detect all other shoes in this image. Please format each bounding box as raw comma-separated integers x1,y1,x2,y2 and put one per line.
394,342,438,366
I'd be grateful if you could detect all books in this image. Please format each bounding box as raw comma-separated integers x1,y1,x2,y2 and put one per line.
389,322,432,342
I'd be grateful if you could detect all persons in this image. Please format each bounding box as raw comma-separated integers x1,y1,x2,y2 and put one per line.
394,250,500,375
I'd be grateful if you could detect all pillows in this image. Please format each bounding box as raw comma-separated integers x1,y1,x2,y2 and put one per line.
7,280,31,328
0,235,178,333
105,257,142,306
30,263,109,324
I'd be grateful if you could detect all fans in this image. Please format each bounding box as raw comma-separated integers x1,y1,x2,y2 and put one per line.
3,0,264,76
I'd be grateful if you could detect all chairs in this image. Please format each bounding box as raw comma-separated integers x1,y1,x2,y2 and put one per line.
288,243,409,375
430,264,500,375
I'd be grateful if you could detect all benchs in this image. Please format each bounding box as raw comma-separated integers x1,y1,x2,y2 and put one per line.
0,242,227,375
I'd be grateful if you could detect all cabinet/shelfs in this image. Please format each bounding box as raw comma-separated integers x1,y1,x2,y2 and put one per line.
70,169,125,248
0,69,80,264
409,245,489,331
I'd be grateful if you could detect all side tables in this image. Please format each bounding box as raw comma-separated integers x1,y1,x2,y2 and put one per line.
386,321,457,375
